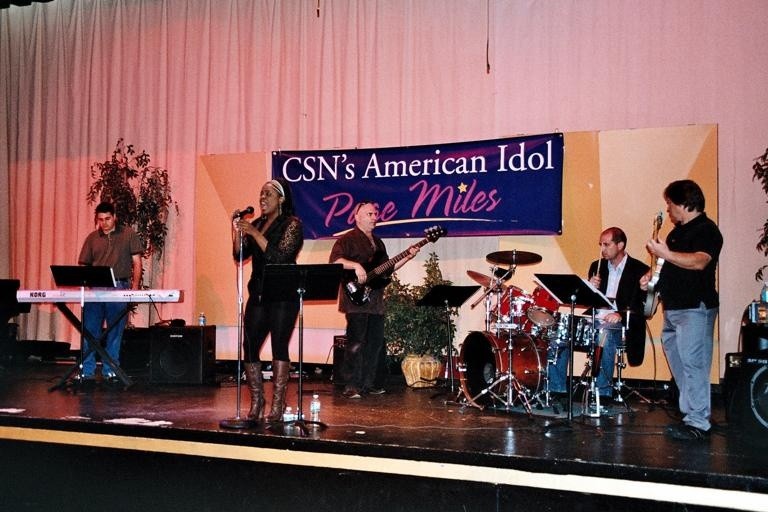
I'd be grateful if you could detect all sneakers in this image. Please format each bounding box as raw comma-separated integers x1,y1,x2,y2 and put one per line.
673,429,702,440
344,391,362,399
664,422,687,434
370,388,385,394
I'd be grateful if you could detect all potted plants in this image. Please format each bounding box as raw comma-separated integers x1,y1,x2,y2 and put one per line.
381,252,459,388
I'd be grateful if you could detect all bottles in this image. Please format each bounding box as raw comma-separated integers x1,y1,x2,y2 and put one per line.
198,313,206,326
294,408,305,436
284,407,294,434
310,395,321,427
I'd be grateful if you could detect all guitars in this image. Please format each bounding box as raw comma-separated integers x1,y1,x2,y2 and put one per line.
643,211,666,319
338,222,446,308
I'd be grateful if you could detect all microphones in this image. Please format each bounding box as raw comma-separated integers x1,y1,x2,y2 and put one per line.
232,207,254,219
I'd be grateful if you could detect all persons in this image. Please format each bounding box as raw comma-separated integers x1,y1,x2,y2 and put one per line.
638,178,724,441
70,201,144,383
329,202,421,400
542,226,650,409
231,177,305,424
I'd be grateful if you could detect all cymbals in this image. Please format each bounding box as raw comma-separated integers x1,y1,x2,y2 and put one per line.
466,269,507,294
485,249,543,267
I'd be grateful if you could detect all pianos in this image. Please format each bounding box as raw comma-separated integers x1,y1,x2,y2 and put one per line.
15,286,182,303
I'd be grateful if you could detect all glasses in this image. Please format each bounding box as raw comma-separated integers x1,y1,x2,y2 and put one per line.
356,201,374,214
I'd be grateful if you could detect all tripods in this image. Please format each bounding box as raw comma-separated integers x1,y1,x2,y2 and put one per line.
464,283,548,413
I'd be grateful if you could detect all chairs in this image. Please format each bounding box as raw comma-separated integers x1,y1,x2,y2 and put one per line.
612,341,655,421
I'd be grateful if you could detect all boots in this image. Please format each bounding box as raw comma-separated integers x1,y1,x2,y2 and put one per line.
244,363,266,420
265,360,289,423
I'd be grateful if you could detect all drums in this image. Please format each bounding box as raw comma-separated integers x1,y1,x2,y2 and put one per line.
531,284,562,312
487,284,539,333
454,328,551,413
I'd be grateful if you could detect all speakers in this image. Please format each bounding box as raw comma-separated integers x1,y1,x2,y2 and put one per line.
146,324,216,391
723,352,768,458
